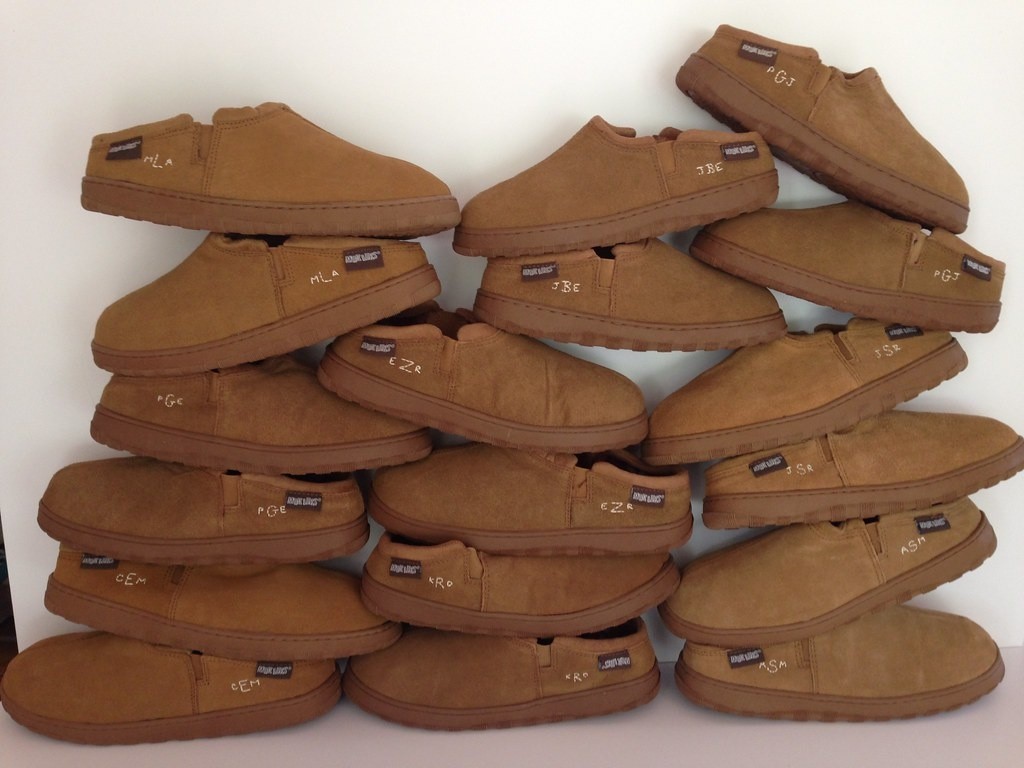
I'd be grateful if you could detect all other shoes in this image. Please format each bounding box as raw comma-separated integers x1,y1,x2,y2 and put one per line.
36,455,370,567
0,630,344,745
691,199,1006,334
701,408,1024,531
473,236,788,352
342,618,662,731
675,603,1005,722
657,497,998,649
90,350,434,476
452,116,779,259
675,25,970,234
318,301,650,451
44,540,400,662
362,532,680,636
79,103,462,239
644,319,968,467
91,229,443,377
367,442,693,559
